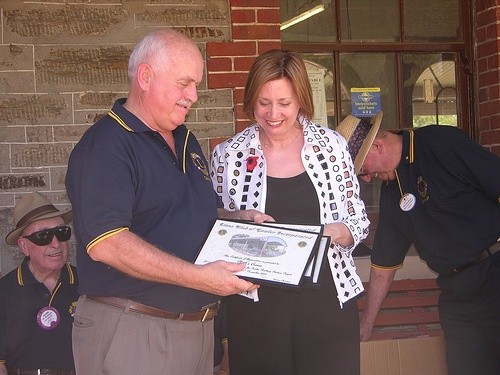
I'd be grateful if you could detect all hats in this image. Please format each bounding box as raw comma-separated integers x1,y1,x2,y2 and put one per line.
5,191,73,245
335,110,383,176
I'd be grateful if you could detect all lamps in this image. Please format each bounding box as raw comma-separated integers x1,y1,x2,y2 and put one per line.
280,0,331,31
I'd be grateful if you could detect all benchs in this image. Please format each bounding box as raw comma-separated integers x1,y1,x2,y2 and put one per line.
357,278,445,343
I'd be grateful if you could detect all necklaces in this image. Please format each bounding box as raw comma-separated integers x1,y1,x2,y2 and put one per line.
259,127,301,149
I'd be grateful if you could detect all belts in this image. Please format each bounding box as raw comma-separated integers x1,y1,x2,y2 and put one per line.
87,294,218,322
441,241,500,277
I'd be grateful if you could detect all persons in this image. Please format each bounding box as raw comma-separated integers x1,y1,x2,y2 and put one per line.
334,109,499,375
1,191,79,375
211,48,371,374
65,30,276,374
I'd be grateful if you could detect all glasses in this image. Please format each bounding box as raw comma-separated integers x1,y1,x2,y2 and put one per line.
22,226,71,246
